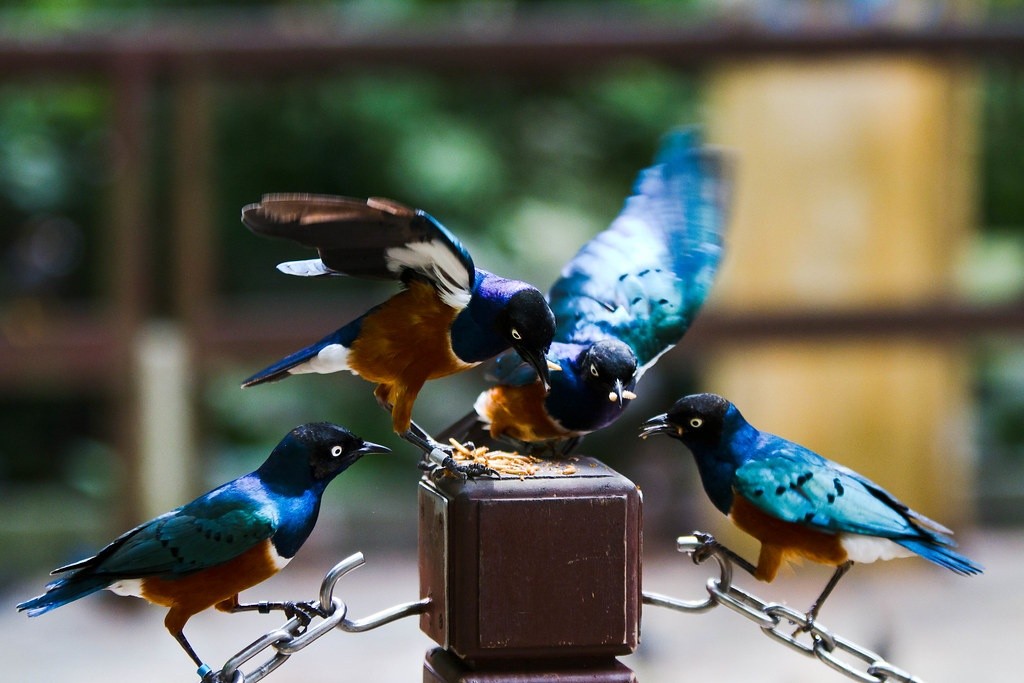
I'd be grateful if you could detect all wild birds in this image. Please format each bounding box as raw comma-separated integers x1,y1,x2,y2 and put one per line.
238,124,729,483
639,393,985,647
16,422,393,683
